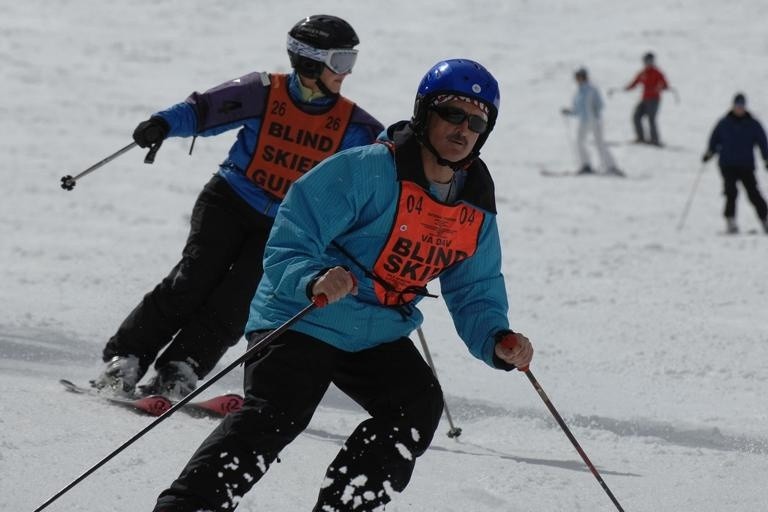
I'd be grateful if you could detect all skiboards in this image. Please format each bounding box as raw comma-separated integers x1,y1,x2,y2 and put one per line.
60,380,245,418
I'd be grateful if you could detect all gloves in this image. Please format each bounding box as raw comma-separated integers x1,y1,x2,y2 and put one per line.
133,115,167,150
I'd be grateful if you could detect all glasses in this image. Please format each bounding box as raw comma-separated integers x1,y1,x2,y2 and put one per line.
427,104,487,135
286,34,358,76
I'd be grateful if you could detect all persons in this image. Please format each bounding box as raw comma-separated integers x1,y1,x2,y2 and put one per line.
151,59,534,512
625,52,668,145
709,94,768,231
92,13,384,404
561,69,624,175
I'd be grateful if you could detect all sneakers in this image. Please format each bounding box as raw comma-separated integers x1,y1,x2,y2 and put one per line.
90,354,139,399
140,359,198,402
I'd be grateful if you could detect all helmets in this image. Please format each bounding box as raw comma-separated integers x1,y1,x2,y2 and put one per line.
413,57,500,140
286,13,360,80
567,48,760,104
